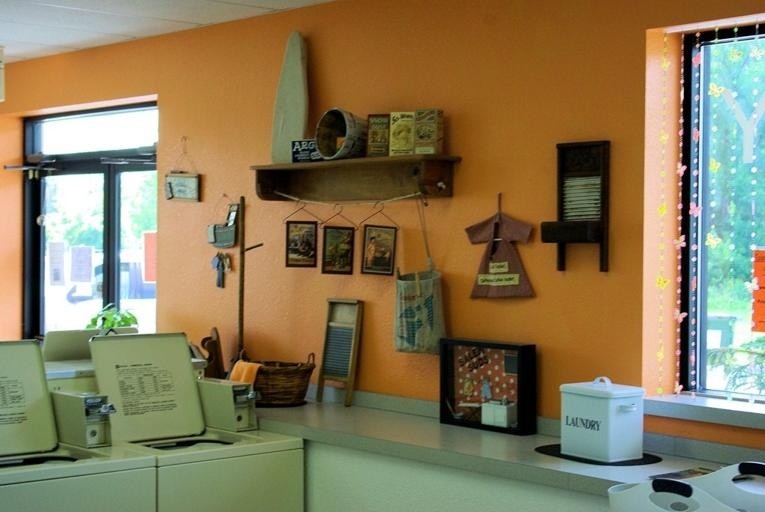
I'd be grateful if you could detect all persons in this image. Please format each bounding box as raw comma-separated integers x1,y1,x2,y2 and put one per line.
365,236,377,272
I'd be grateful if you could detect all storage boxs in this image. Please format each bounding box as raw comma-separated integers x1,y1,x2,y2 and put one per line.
290,104,448,164
558,376,647,465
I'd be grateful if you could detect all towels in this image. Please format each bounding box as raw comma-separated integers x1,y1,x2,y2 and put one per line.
227,359,263,392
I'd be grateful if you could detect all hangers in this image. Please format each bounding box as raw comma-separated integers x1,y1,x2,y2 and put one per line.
361,199,400,233
282,197,322,224
321,203,360,230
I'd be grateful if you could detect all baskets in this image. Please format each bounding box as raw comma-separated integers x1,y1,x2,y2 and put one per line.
234,348,317,406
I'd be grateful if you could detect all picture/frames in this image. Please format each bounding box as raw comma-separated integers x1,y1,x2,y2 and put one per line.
284,219,320,269
360,223,397,279
320,225,357,276
